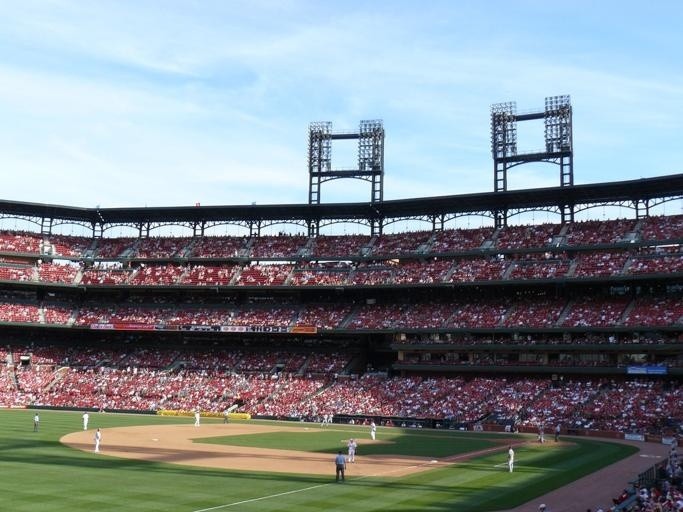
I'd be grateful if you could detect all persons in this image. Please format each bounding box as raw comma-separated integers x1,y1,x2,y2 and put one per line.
94,428,101,452
0,215,683,286
0,290,681,370
508,446,514,473
0,365,682,443
335,439,356,481
609,435,682,511
